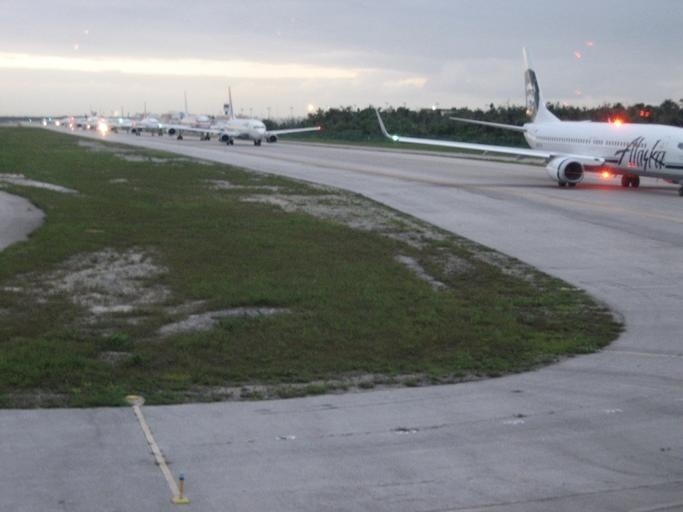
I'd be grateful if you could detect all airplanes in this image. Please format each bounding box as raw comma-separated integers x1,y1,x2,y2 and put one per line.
41,108,321,148
373,46,682,197
